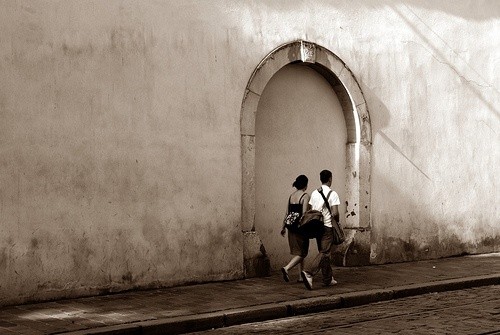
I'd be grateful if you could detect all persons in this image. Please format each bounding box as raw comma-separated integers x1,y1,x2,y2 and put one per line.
300,170,341,291
279,174,310,283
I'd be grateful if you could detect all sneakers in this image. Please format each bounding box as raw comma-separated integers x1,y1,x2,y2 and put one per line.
280,267,290,282
327,277,337,286
301,270,313,290
297,278,304,282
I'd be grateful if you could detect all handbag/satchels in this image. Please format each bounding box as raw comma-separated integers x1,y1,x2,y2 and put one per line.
283,212,299,229
332,218,346,245
297,209,324,239
